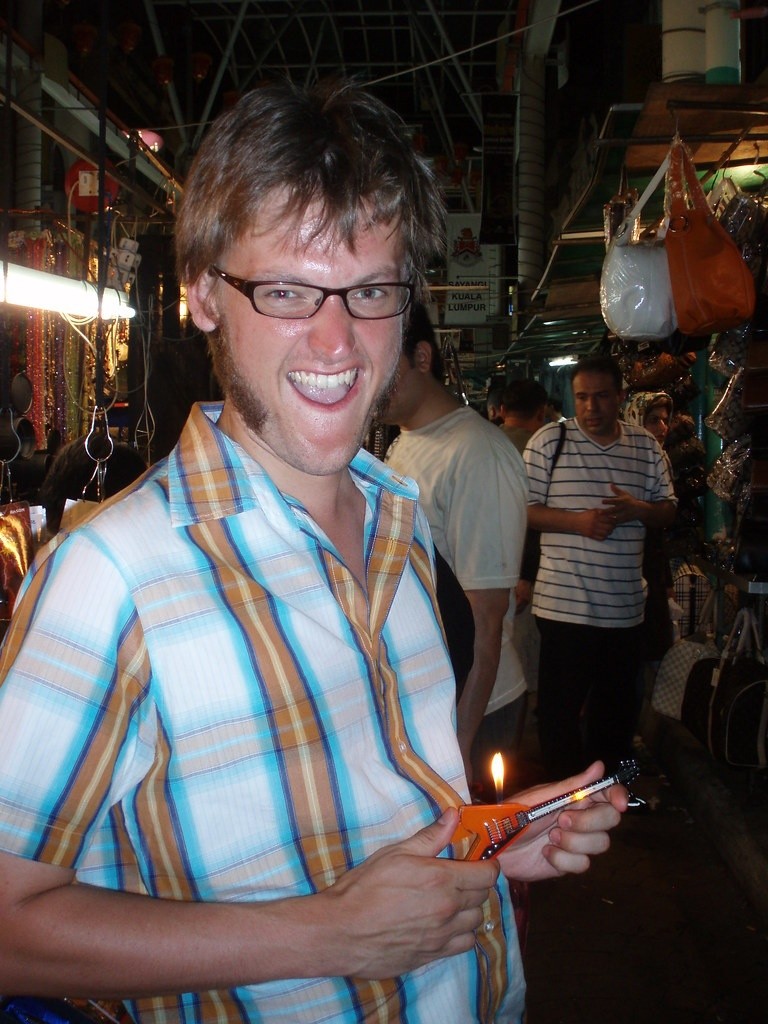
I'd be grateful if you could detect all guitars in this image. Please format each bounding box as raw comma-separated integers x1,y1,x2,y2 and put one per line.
461,760,650,862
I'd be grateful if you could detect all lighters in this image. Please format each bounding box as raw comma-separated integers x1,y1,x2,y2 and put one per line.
449,759,639,863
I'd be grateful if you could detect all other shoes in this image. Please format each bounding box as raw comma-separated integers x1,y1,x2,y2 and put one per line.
623,793,649,814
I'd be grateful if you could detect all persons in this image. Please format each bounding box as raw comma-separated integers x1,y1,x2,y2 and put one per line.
543,392,568,423
523,357,680,812
376,304,530,806
623,391,674,753
39,433,148,534
1,80,626,1024
486,376,509,426
499,379,547,613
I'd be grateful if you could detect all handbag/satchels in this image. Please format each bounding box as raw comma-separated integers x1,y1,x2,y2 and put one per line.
598,131,765,771
519,526,543,581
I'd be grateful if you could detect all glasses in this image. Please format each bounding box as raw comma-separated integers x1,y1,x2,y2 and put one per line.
213,264,415,320
